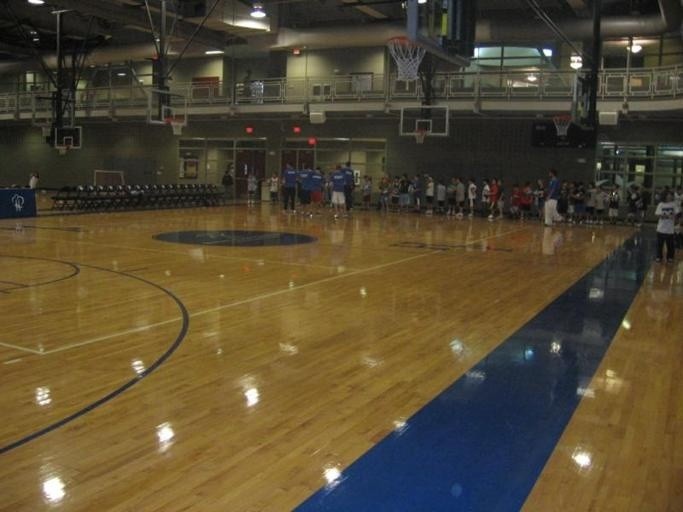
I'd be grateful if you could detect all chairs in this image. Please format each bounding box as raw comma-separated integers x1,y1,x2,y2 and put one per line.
50,183,225,214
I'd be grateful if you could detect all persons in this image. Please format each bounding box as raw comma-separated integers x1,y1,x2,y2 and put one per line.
221,169,233,196
644,266,676,333
281,162,354,217
652,188,680,265
265,172,281,204
244,170,263,210
540,225,566,274
543,168,682,227
361,172,548,222
